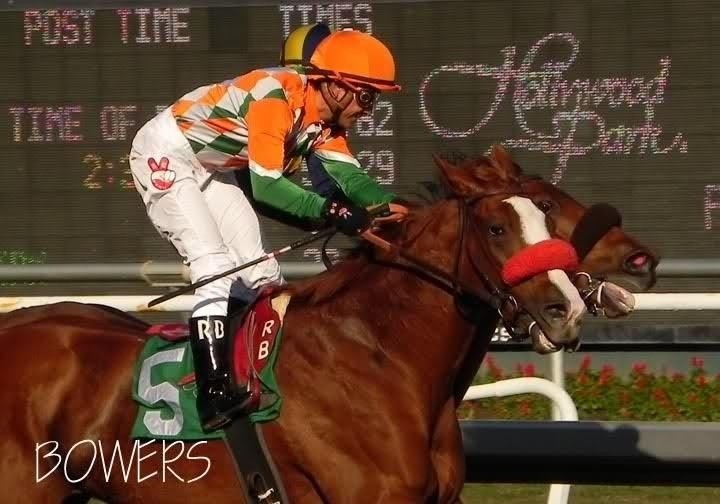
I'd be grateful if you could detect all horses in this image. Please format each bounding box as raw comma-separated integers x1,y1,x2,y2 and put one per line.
0,138,593,504
427,141,662,504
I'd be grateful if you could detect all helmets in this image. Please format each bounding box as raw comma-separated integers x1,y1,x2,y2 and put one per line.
280,22,330,67
310,28,401,91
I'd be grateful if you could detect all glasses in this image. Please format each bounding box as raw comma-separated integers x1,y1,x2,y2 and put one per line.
309,62,382,110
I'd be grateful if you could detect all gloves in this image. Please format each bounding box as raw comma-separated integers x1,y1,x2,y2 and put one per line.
321,190,371,236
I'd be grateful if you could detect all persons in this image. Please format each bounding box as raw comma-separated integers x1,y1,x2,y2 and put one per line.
128,21,407,432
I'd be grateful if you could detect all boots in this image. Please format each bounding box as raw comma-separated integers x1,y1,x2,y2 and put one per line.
188,316,253,431
